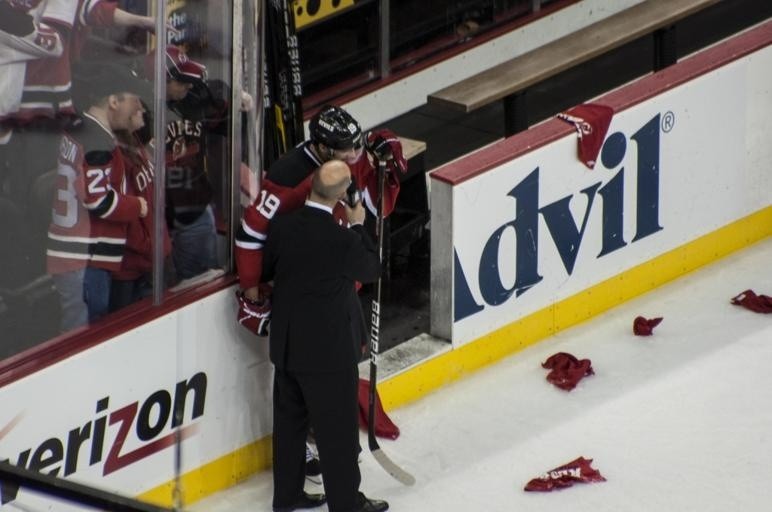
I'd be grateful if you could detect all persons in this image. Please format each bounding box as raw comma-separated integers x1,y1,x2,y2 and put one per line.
232,104,409,476
259,158,390,512
1,1,256,339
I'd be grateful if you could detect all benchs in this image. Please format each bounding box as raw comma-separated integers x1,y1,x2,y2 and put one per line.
426,3,718,138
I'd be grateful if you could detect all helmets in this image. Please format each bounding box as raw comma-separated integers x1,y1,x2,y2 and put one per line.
307,101,364,153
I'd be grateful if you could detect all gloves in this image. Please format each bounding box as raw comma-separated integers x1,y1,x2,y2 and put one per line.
364,128,408,176
234,280,273,338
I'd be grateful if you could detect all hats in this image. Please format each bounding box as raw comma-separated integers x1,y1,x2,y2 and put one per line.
85,62,154,99
143,43,208,83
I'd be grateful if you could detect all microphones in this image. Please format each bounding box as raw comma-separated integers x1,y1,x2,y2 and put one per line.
346,174,357,208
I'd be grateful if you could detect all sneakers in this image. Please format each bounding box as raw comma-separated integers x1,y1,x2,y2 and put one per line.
303,438,322,478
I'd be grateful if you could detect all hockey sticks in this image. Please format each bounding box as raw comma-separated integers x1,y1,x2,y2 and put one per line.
369,156,416,486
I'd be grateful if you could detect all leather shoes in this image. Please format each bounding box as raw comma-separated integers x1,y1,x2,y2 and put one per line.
272,490,326,512
359,493,388,512
